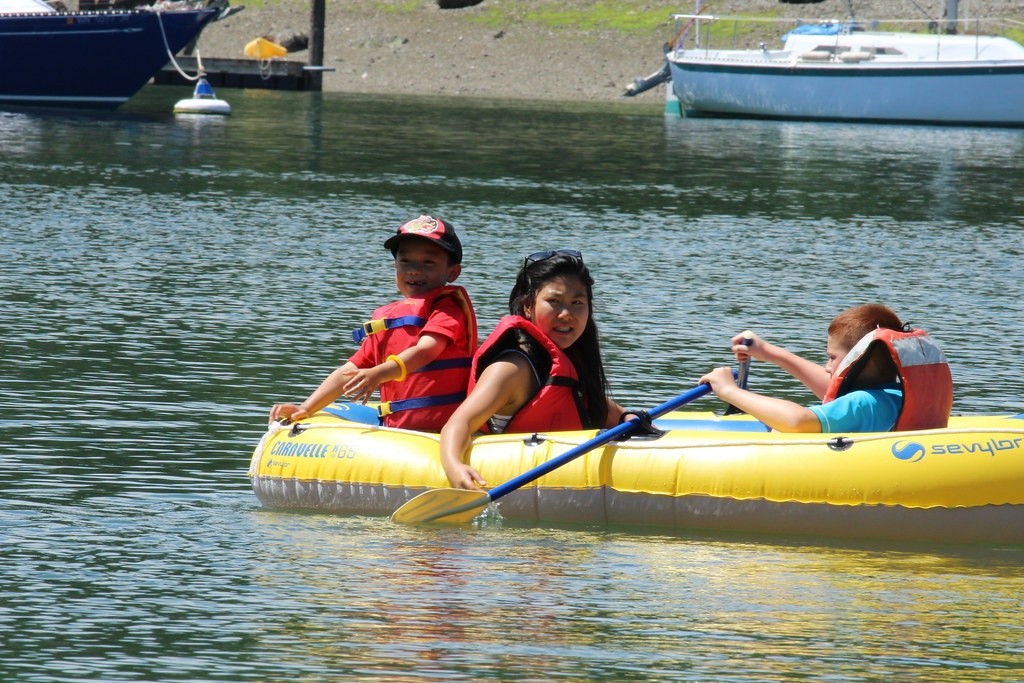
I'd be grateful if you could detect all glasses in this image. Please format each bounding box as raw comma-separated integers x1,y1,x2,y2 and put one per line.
524,250,583,281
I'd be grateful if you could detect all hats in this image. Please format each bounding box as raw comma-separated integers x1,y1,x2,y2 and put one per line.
384,215,462,264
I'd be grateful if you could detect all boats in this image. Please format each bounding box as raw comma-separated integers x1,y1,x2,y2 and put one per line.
621,2,1023,131
242,36,288,60
243,386,1024,555
0,0,230,110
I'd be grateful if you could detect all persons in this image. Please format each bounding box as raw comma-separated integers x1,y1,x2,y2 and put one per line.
699,303,903,432
441,250,637,489
268,214,478,423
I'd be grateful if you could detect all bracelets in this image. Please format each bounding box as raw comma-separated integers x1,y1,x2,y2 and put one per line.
619,411,636,425
386,355,405,381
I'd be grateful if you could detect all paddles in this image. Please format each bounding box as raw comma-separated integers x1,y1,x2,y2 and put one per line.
392,372,734,526
725,329,754,417
